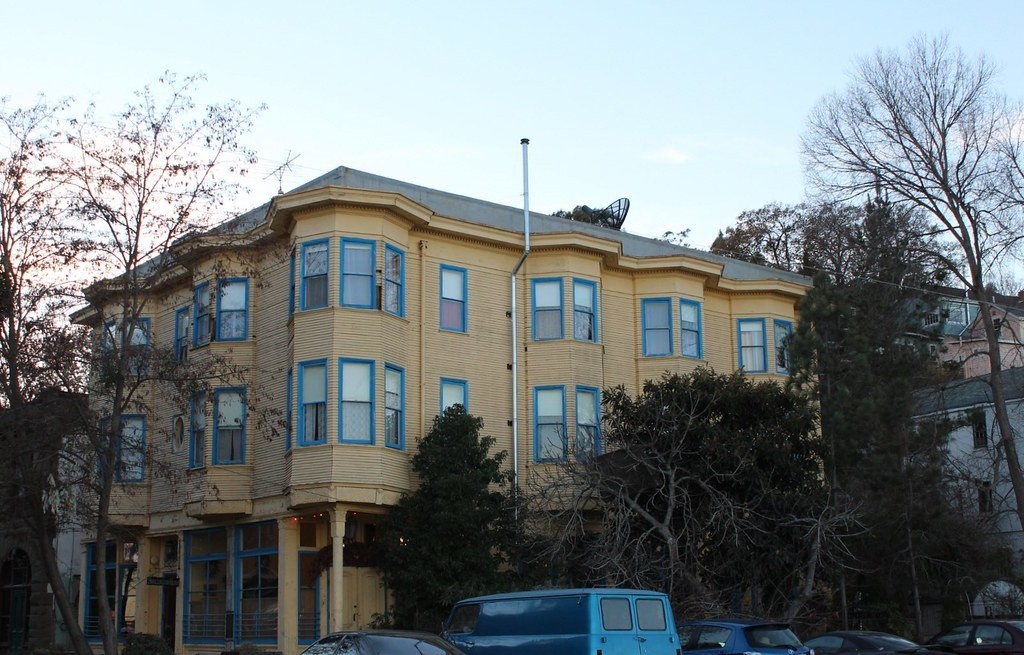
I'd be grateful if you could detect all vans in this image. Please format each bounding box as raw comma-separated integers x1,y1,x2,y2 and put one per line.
437,587,683,655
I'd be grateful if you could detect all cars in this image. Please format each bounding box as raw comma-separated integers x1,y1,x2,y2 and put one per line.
801,630,958,655
675,618,816,655
921,618,1024,655
298,629,467,655
